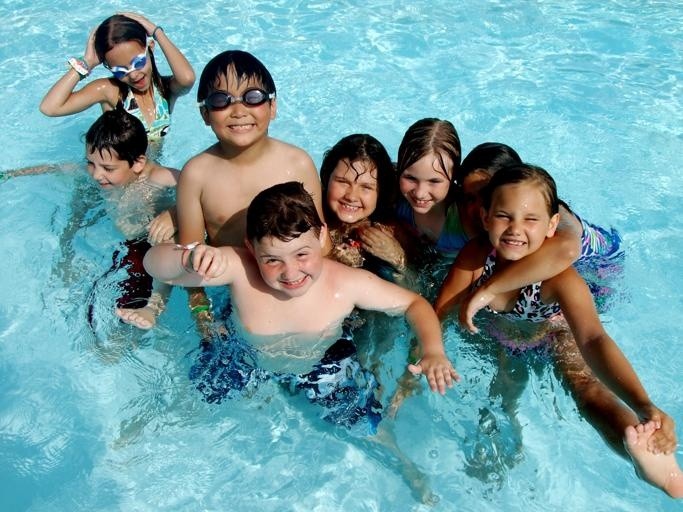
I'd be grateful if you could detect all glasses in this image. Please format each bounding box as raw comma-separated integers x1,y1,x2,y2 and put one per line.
102,33,148,80
197,87,275,112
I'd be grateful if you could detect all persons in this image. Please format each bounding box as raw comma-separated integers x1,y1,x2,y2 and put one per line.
38,10,197,281
116,179,459,503
321,116,682,498
0,108,182,365
174,50,323,361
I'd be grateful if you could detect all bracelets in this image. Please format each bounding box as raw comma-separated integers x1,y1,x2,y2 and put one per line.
66,56,91,81
152,25,163,40
175,241,202,274
186,291,211,319
